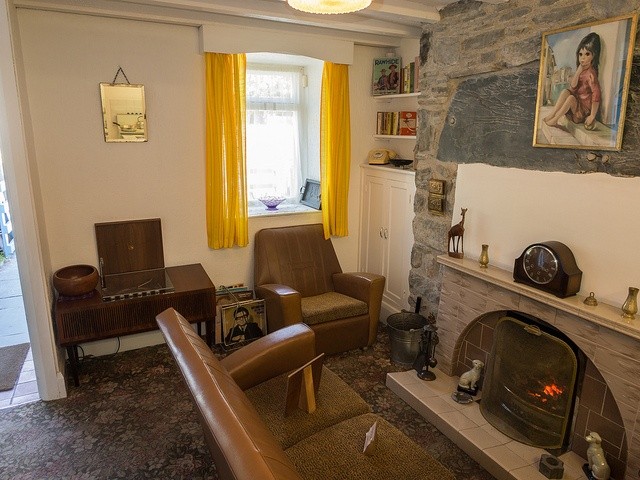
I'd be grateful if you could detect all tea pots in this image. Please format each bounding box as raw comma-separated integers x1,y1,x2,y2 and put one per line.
136,115,144,129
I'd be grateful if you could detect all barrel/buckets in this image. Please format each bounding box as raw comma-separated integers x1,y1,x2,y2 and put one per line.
385,309,429,367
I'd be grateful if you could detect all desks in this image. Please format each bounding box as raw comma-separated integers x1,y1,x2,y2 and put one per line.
51,263,222,388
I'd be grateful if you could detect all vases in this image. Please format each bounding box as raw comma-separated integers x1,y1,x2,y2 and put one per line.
480,242,492,270
621,284,640,322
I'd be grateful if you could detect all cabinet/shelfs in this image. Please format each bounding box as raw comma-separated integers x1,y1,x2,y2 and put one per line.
361,161,416,326
375,92,421,140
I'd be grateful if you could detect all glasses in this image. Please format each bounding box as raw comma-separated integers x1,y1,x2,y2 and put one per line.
235,315,248,320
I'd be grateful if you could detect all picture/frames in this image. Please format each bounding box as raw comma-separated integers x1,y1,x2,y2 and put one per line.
300,178,322,210
532,11,640,153
285,352,327,417
372,54,403,96
214,282,269,355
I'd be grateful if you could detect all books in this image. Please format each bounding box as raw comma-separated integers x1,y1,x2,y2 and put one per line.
401,56,420,93
375,111,416,136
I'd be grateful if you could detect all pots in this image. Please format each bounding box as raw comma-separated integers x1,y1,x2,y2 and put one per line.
112,121,135,133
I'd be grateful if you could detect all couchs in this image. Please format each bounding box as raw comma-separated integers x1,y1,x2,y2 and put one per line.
254,223,387,358
153,306,458,478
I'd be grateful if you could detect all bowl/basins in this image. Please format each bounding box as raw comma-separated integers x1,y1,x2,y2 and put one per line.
388,159,414,168
52,264,100,298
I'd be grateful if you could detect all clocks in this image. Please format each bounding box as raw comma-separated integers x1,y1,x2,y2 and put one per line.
523,246,561,286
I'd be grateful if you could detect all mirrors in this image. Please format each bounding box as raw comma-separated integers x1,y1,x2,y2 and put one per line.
98,81,149,143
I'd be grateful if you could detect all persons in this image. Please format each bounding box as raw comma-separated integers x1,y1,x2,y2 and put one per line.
387,64,399,90
377,68,388,89
542,32,602,127
226,307,263,344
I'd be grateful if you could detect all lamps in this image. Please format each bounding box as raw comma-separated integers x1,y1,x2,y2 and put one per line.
288,1,373,14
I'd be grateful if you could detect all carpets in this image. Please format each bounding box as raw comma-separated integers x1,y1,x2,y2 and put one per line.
0,321,493,479
0,342,31,392
